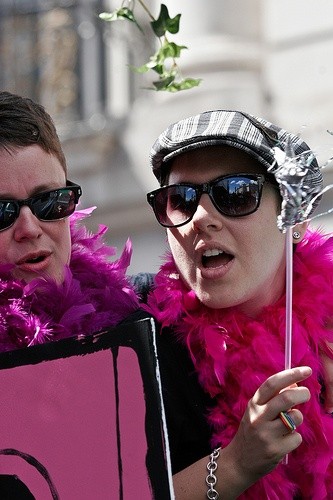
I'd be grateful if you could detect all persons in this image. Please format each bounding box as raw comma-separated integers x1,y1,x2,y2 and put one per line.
0,87,208,500
153,110,333,500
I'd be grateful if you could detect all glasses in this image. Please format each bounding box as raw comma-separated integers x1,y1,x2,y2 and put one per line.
146,171,269,228
0,180,81,232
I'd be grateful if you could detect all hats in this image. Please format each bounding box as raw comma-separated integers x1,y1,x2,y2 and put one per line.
149,110,322,218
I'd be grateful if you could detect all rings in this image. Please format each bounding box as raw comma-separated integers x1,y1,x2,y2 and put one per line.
277,409,297,433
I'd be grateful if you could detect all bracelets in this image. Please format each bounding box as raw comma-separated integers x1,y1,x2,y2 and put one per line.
206,446,223,500
